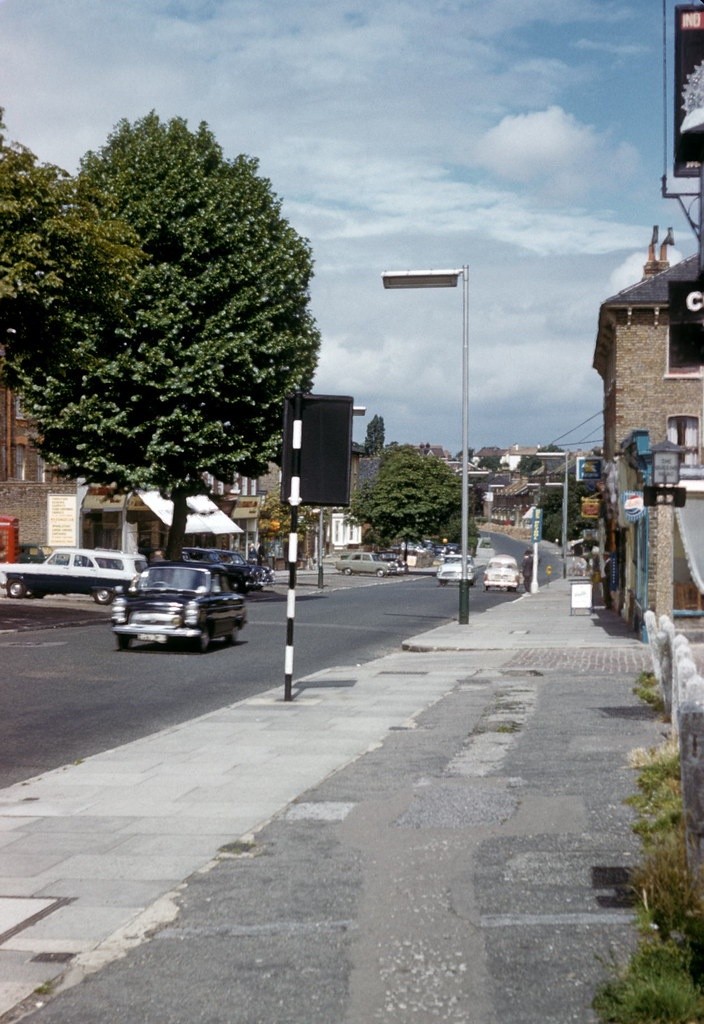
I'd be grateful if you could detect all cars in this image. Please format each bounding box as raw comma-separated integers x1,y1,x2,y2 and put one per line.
1,544,275,605
112,567,248,653
376,552,408,576
374,538,460,560
483,553,525,592
336,551,397,577
437,554,478,586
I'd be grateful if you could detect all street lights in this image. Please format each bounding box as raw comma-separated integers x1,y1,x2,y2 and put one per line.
381,264,471,624
537,450,568,578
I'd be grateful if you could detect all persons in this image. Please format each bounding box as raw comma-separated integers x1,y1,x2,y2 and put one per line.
247,540,266,566
521,550,534,597
601,552,615,610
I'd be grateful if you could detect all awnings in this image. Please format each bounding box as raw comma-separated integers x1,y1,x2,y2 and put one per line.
134,488,245,535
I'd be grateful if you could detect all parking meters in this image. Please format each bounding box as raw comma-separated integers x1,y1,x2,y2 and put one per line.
545,566,551,588
